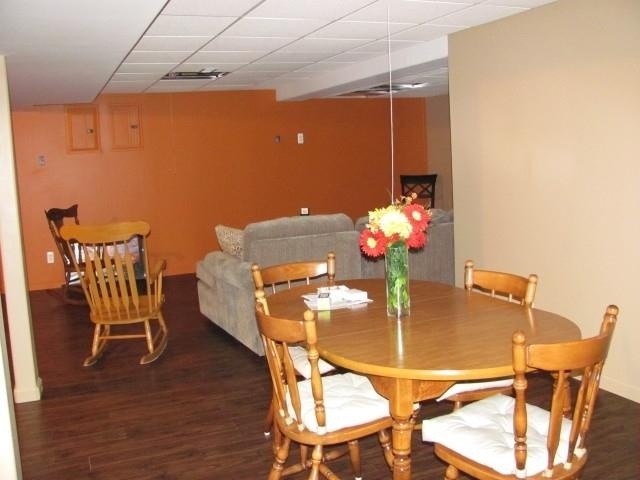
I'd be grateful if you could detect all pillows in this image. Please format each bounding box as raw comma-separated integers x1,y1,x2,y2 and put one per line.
355,207,449,233
215,224,243,260
447,208,453,222
243,213,355,262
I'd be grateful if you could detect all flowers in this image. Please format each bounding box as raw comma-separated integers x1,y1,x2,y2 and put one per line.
360,193,432,258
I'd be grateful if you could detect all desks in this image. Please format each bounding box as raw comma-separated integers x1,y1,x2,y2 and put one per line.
266,279,581,480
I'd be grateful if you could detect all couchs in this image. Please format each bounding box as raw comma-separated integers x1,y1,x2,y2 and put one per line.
194,223,454,357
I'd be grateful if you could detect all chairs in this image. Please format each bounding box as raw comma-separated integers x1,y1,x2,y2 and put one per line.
422,305,619,480
45,204,143,304
251,251,337,436
435,259,538,411
60,220,168,366
253,291,419,480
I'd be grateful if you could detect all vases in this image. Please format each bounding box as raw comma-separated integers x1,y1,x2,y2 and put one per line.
383,246,410,317
